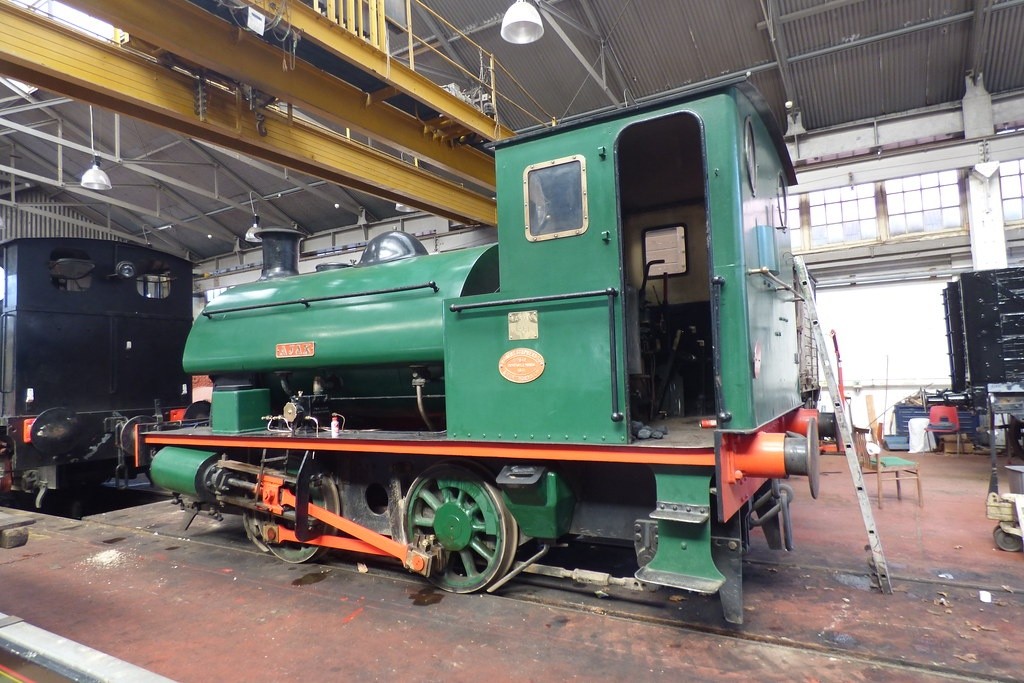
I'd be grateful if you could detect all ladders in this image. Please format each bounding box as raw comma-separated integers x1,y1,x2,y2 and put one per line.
794,255,892,593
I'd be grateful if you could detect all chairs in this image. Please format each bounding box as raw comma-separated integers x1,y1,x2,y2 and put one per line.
851,425,924,510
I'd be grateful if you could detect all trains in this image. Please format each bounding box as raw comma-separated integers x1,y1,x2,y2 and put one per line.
0,63,820,625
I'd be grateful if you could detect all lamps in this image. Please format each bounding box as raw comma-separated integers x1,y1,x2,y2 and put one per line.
246,190,267,243
81,101,114,190
500,0,544,44
